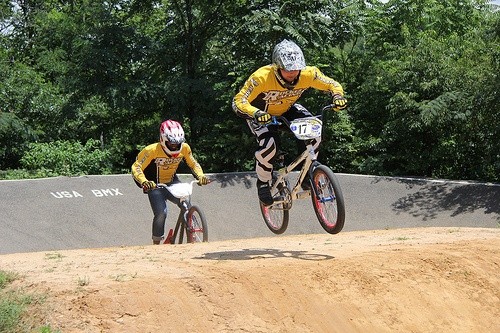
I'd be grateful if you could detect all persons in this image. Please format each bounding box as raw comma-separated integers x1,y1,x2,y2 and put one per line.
131,120,207,245
232,39,347,206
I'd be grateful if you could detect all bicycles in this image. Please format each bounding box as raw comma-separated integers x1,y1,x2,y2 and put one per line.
142,181,212,243
256,104,346,234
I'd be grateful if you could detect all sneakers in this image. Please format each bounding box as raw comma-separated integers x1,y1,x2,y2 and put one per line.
257,178,274,205
302,177,321,190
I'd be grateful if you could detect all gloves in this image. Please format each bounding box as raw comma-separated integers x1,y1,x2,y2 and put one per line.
143,181,157,191
332,94,349,110
255,109,271,125
198,176,209,186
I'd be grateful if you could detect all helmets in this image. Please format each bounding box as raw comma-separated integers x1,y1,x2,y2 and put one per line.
159,119,186,159
272,38,306,91
